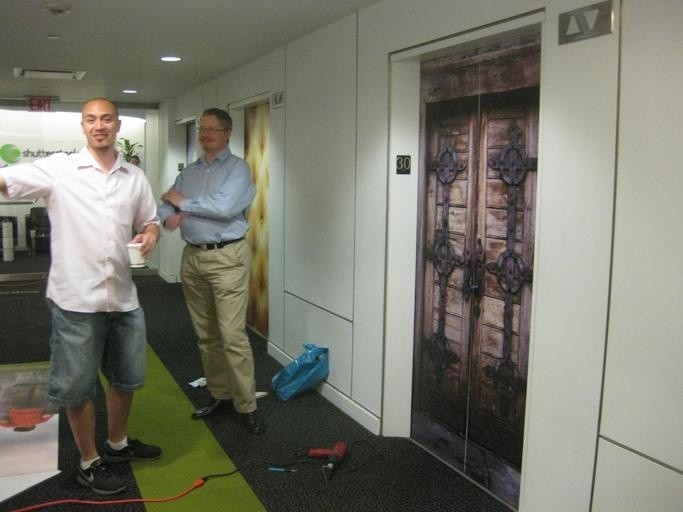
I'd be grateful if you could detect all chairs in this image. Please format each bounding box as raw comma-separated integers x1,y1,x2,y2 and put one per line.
24,207,50,256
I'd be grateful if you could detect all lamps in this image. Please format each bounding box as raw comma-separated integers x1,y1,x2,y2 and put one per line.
21,69,76,81
41,4,72,16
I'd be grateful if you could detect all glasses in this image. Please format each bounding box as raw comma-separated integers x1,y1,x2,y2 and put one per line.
196,126,226,134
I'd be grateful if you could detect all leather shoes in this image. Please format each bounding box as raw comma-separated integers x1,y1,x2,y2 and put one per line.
240,407,265,434
191,396,235,420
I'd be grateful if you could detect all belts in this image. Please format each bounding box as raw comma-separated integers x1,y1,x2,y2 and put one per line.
183,235,246,250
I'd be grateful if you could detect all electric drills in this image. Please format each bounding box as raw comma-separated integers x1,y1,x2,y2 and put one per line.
308,440,349,482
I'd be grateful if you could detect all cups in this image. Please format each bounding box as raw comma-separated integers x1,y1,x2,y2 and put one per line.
125,243,146,269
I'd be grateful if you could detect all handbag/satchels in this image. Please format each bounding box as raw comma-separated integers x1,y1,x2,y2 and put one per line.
269,339,329,404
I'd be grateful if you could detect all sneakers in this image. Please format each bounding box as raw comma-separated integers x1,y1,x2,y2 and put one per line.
76,458,126,497
103,434,163,464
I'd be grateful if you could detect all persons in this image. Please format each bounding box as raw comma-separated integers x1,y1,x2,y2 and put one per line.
158,107,266,435
0,97,161,495
128,155,140,167
